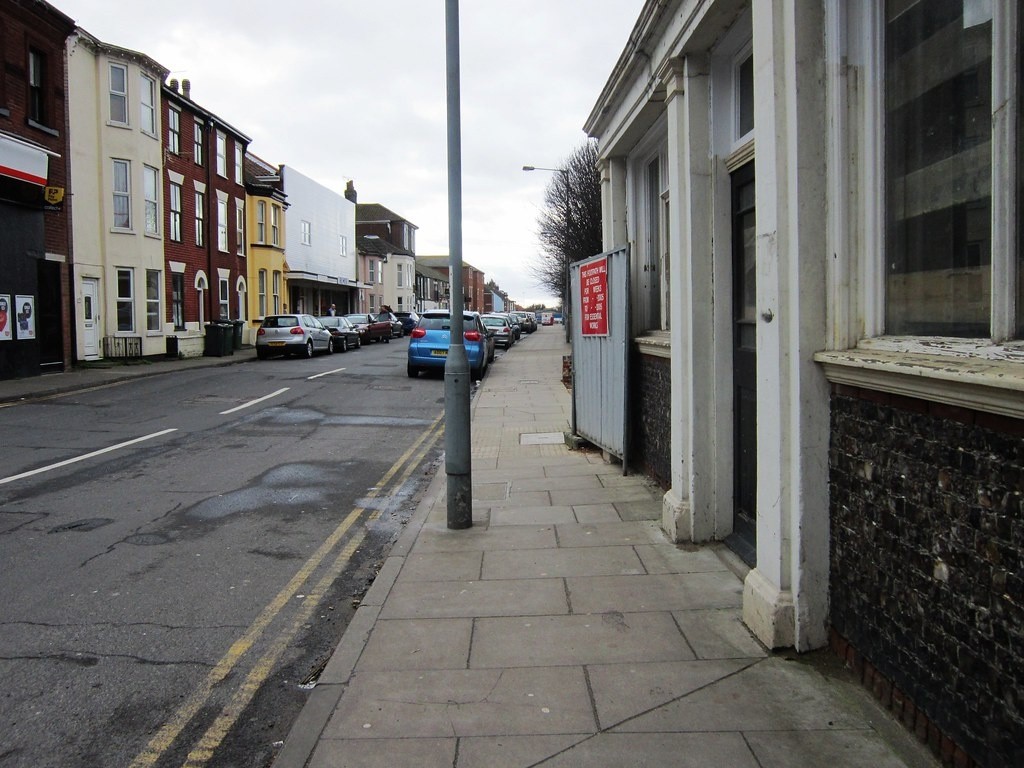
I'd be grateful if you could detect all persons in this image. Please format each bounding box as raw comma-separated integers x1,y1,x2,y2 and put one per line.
326,304,337,316
372,306,391,343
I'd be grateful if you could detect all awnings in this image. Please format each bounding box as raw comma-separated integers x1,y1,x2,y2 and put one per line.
0,133,48,187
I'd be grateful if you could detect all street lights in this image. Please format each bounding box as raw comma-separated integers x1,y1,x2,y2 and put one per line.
523,166,569,344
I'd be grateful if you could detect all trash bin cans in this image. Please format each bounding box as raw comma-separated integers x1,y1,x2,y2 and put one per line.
204,323,234,356
213,319,245,348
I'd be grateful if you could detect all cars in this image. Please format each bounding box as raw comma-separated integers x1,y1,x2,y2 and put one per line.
316,316,361,353
480,311,537,348
256,314,334,358
408,309,495,379
343,311,419,344
